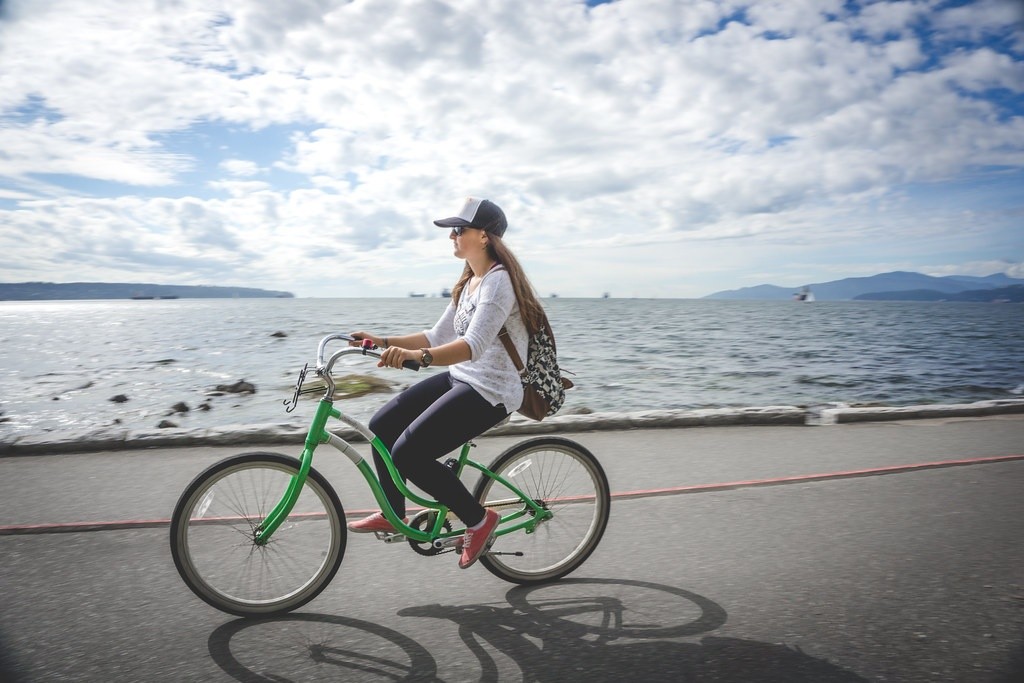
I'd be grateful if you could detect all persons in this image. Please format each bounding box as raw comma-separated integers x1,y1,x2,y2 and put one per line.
347,196,574,569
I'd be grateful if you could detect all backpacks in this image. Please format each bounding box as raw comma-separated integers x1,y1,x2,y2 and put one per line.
457,268,576,422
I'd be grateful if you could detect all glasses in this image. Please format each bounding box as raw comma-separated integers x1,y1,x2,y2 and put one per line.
453,226,465,235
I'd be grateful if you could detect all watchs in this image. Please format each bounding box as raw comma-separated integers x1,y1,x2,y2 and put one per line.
419,348,433,368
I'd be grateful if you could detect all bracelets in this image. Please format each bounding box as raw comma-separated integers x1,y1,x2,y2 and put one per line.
382,338,389,350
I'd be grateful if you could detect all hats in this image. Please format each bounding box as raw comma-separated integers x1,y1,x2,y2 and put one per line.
433,197,507,238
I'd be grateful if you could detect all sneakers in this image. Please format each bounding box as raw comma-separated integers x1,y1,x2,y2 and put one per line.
459,508,501,569
347,511,410,533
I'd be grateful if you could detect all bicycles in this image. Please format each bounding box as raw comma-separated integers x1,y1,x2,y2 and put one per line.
169,332,610,617
